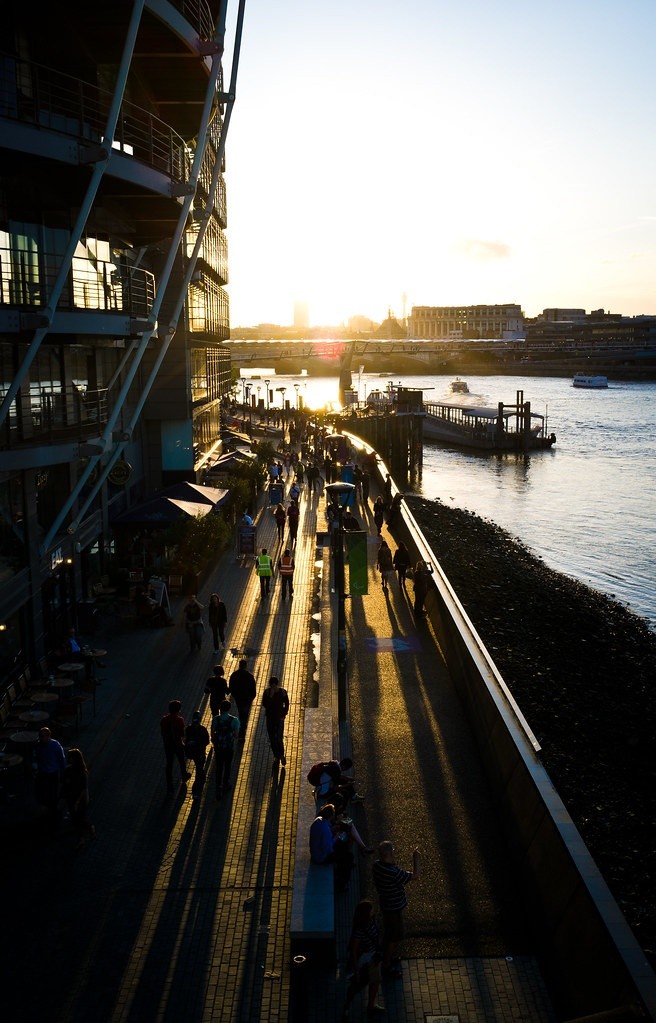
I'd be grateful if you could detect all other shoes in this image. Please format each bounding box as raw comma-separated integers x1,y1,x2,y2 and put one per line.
367,1001,386,1011
272,755,287,770
192,786,203,796
222,642,226,647
180,773,192,782
192,645,201,651
216,782,232,791
416,611,425,617
212,649,221,657
282,595,293,601
344,1007,352,1018
262,591,271,600
168,787,174,794
362,846,376,858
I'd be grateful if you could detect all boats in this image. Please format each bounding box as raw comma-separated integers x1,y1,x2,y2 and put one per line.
368,390,557,452
451,382,470,393
572,375,609,388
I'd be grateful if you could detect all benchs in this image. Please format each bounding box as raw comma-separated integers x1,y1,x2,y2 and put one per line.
316,496,329,545
290,708,335,983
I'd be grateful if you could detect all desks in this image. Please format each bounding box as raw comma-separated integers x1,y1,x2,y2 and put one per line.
46,648,107,703
0,693,59,804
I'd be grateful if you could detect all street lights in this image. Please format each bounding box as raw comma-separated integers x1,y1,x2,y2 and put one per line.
280,387,286,433
241,377,245,403
294,384,300,410
247,382,253,442
264,379,270,425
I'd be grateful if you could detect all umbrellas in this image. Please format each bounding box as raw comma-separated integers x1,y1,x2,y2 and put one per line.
113,480,230,565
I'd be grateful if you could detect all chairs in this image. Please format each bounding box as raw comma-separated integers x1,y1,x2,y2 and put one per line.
53,680,97,736
132,594,163,629
0,656,56,752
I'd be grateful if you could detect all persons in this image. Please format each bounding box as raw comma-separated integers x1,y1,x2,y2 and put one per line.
211,700,241,790
262,677,289,765
372,841,421,951
229,660,256,742
59,628,106,686
343,901,390,1016
132,584,173,628
182,594,206,655
160,700,191,784
307,757,374,893
209,593,228,654
203,666,231,717
219,396,434,617
185,711,209,799
29,727,96,834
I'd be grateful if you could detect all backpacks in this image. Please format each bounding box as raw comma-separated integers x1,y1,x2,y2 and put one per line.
216,724,234,751
307,763,325,786
